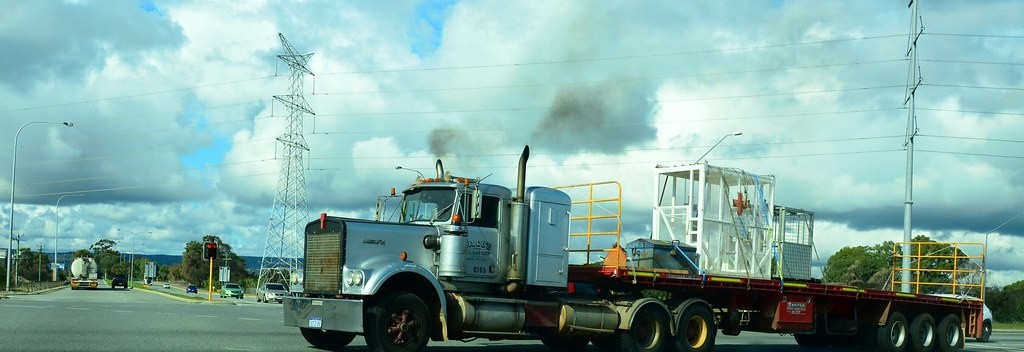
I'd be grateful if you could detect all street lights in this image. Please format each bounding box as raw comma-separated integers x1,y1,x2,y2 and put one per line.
118,227,153,291
35,241,46,283
11,231,23,285
5,118,74,292
51,192,85,282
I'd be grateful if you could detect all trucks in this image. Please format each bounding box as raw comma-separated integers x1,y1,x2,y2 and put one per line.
70,254,98,290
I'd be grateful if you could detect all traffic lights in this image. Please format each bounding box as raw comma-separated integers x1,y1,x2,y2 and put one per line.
203,242,212,261
212,242,218,262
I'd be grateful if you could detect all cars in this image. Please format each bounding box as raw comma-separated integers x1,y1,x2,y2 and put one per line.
256,281,291,304
186,283,197,293
163,283,171,289
219,283,244,300
925,290,994,344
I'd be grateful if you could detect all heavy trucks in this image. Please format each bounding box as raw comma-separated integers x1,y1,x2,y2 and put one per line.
281,145,988,351
